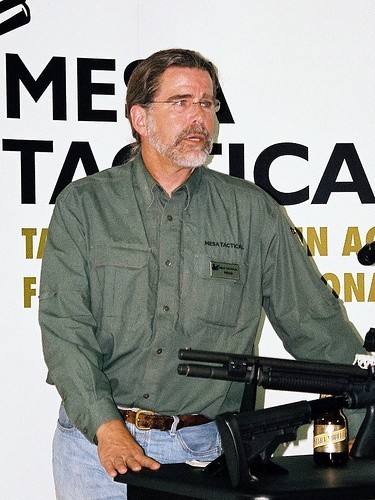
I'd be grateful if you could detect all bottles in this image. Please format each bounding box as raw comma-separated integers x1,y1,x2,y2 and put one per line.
313,393,349,468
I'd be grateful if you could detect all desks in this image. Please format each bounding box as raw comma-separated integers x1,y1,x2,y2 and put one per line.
114,452,375,500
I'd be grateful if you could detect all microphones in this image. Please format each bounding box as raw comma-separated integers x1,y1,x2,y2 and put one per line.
358,241,375,265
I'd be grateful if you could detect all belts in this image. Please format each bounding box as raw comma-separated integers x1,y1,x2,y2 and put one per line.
117,408,214,431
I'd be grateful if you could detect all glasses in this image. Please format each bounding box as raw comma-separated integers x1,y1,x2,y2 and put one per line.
127,97,221,117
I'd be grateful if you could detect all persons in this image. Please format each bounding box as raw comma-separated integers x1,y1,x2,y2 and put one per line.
38,49,375,500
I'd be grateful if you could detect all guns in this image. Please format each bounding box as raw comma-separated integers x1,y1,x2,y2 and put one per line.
178,326,375,489
